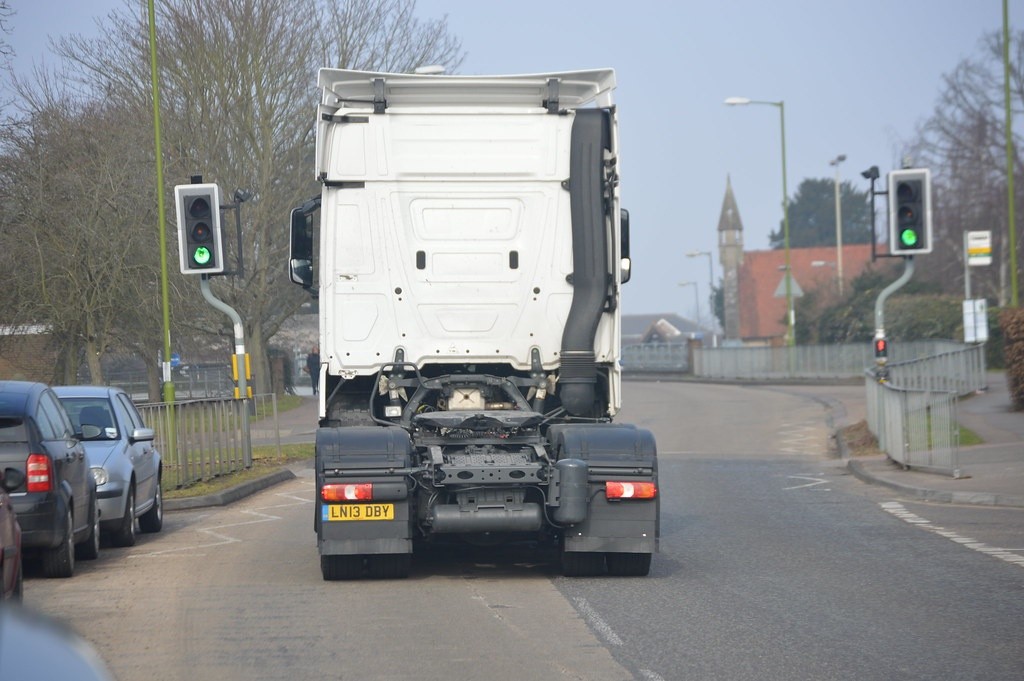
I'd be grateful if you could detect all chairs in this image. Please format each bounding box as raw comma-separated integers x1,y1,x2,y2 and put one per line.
79,405,109,438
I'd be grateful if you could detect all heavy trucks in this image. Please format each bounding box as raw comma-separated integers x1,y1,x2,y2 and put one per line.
285,66,663,583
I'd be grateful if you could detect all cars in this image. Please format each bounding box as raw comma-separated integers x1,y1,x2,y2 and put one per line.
0,380,101,579
51,383,164,547
0,466,28,608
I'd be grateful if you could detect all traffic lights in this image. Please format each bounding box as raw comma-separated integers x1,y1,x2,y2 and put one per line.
174,183,226,274
888,169,934,257
876,339,887,358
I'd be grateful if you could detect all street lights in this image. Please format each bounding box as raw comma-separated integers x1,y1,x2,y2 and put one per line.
688,251,714,292
681,281,701,329
727,93,795,345
829,153,848,296
